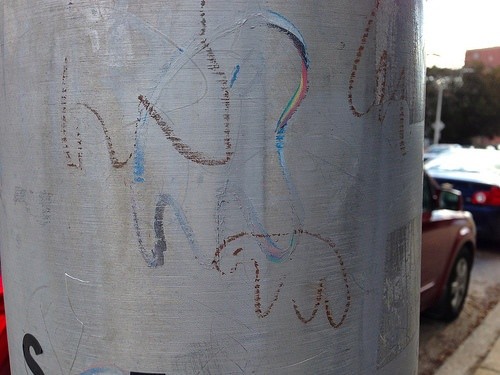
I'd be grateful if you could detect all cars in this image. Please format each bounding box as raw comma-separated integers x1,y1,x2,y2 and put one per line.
423,149,499,249
423,144,461,162
421,165,477,323
465,144,500,150
